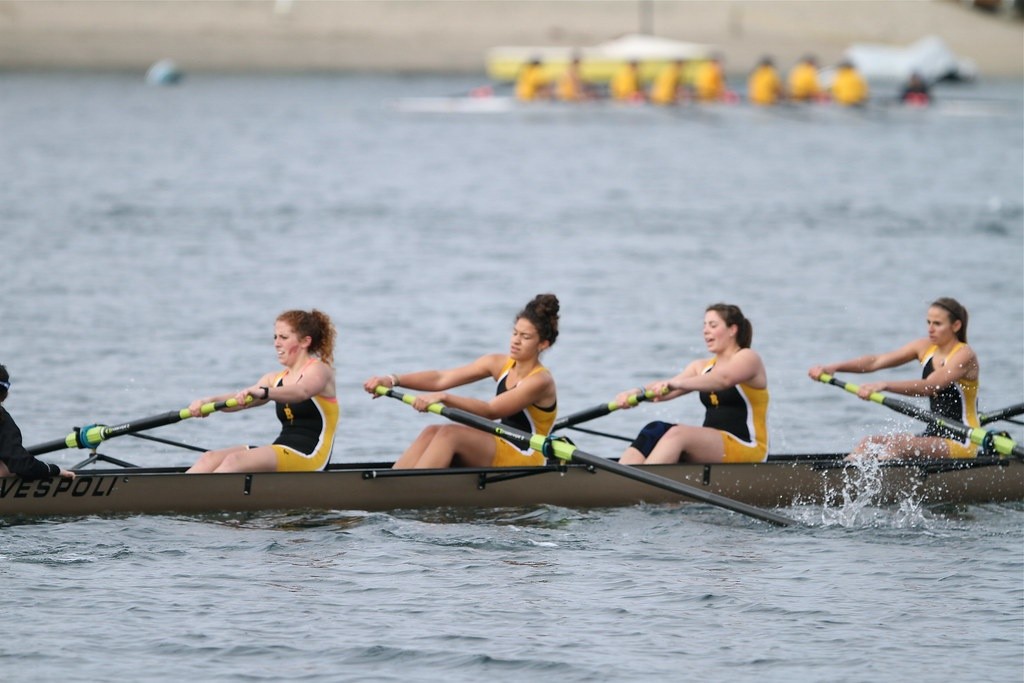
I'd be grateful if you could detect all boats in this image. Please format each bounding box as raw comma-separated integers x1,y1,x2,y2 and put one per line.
395,90,991,120
1,445,1022,524
823,36,988,96
487,36,727,91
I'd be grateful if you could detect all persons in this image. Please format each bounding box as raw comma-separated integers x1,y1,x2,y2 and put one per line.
185,308,341,472
807,297,982,465
615,301,770,465
514,53,938,114
361,292,559,471
0,364,77,481
